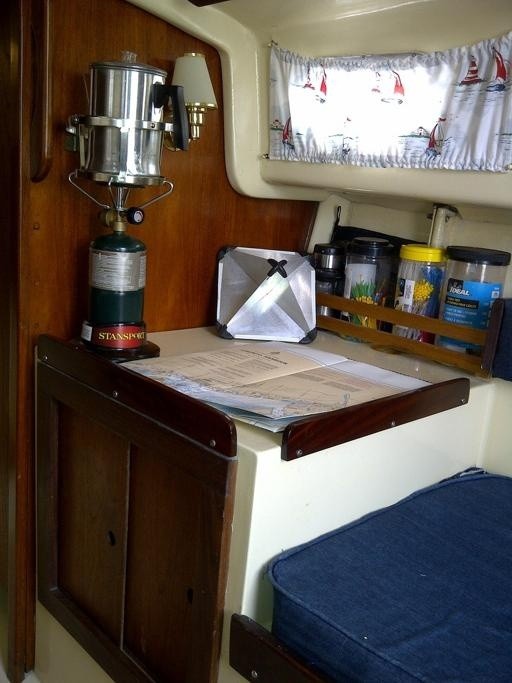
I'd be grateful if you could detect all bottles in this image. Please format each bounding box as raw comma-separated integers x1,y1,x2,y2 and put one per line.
313,237,512,379
85,221,147,359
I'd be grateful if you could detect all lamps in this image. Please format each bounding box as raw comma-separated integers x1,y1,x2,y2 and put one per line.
161,51,220,152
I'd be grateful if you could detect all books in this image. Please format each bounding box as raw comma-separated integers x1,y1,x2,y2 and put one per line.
121,340,434,434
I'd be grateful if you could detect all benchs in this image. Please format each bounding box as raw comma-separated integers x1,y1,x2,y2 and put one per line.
230,462,511,683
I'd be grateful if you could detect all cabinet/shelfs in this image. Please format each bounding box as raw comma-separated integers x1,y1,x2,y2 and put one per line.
37,323,471,683
